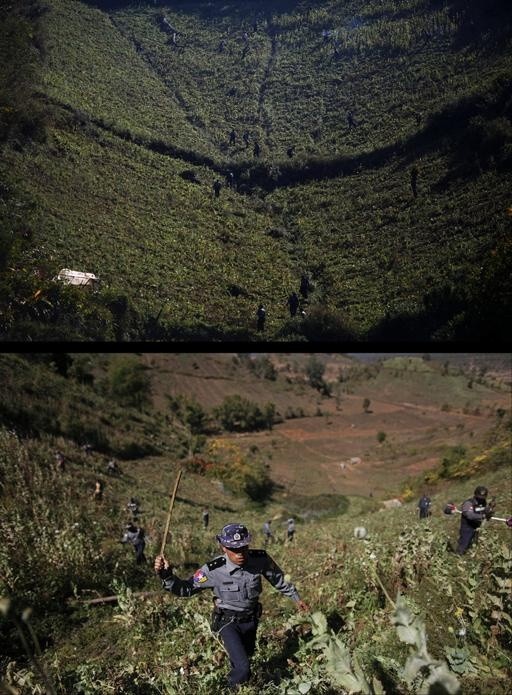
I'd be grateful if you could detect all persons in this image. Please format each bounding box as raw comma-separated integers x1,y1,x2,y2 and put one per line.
243,131,251,149
128,496,142,523
96,479,104,500
55,449,66,474
213,179,222,199
299,276,309,300
153,522,313,691
417,492,433,520
203,503,212,531
262,520,275,547
253,142,261,160
287,515,297,543
287,144,296,158
454,486,498,557
347,111,357,132
256,304,266,335
227,130,240,148
284,292,300,319
121,522,148,566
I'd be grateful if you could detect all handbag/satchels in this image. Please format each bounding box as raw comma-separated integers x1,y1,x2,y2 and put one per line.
211,607,222,631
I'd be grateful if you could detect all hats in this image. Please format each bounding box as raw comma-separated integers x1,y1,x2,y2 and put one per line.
475,486,487,499
216,524,250,549
